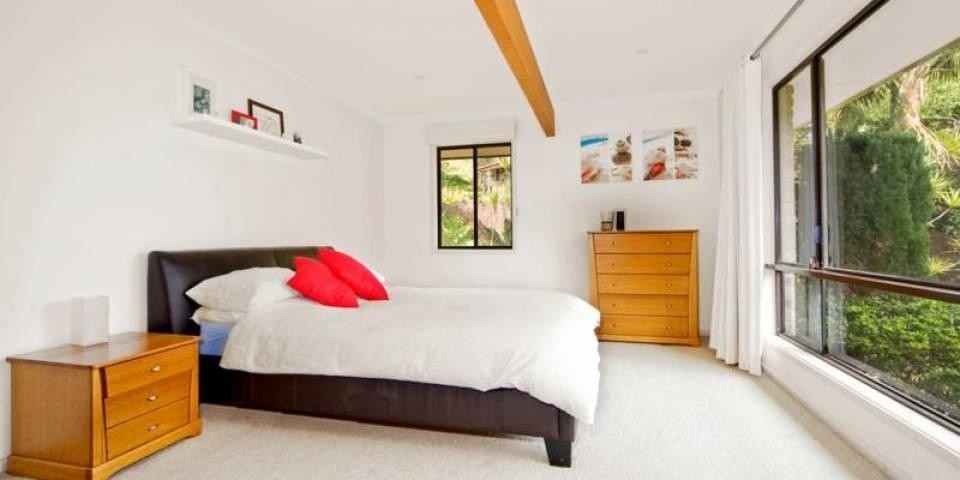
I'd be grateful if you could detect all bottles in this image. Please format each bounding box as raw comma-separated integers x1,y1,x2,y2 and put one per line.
616,207,625,231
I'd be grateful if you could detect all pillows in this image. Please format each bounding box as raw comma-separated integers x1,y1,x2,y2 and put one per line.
181,246,391,311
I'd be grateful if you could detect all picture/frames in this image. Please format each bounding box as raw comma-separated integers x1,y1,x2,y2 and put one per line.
231,95,288,141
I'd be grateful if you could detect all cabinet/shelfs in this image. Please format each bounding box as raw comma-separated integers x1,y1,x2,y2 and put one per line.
2,329,205,480
585,225,705,349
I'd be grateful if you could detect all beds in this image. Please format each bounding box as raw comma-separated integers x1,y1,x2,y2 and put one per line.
145,240,604,470
